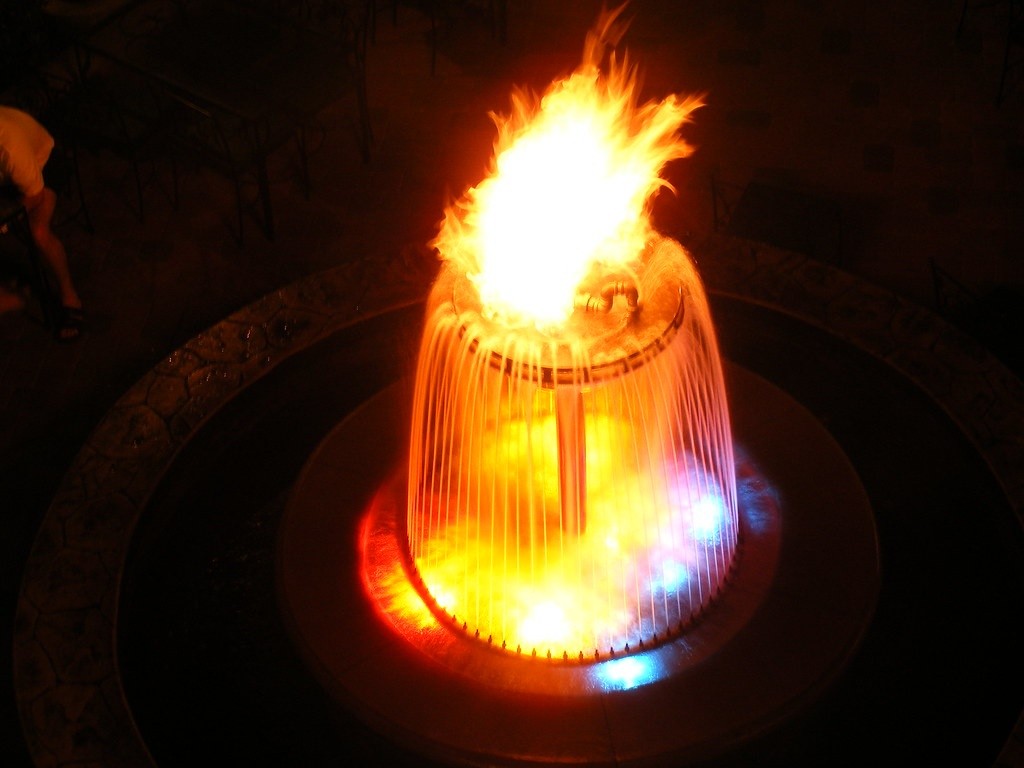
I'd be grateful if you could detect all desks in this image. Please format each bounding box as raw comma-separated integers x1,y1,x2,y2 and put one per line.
73,0,371,240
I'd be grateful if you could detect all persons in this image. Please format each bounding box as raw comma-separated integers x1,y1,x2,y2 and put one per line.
1,104,86,344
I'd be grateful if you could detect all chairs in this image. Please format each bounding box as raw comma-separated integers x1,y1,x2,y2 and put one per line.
280,0,378,166
24,38,206,224
146,75,311,248
9,63,97,248
